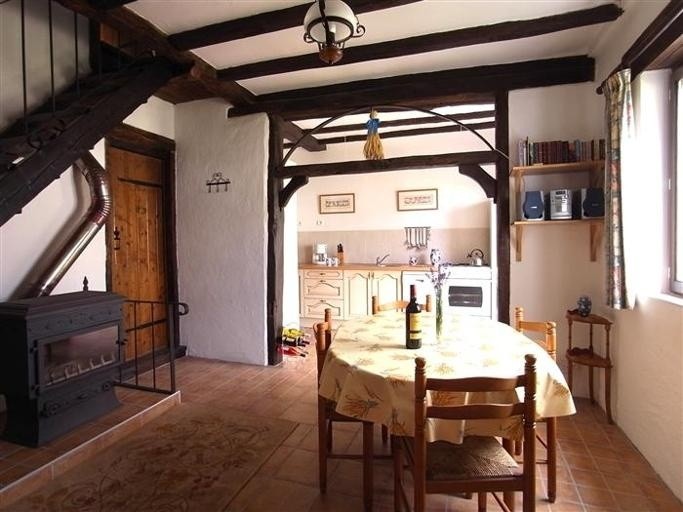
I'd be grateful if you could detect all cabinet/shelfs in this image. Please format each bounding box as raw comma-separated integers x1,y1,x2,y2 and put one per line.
566,311,612,424
343,270,402,322
298,270,343,329
510,160,605,262
402,272,438,311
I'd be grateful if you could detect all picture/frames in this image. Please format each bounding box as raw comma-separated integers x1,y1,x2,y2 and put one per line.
319,193,355,214
397,189,439,211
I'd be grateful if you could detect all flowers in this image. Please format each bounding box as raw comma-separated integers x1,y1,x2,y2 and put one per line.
425,261,452,336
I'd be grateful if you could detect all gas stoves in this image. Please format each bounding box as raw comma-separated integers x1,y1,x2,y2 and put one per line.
438,262,489,267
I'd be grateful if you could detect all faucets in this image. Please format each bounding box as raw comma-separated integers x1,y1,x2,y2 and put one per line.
376,253,389,265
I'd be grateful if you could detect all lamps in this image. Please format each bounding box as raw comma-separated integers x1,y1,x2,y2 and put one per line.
303,0,365,66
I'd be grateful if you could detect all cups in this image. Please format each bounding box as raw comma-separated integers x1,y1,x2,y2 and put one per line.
326,257,339,268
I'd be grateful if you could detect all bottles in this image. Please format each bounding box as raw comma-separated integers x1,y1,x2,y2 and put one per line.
335,243,344,266
280,326,311,357
404,283,423,350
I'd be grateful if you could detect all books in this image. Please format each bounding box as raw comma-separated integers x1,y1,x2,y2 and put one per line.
516,137,605,166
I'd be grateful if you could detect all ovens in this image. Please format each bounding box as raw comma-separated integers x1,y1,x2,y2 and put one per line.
440,278,491,318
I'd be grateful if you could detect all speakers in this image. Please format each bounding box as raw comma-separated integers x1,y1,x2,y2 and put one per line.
521,191,545,221
581,187,605,219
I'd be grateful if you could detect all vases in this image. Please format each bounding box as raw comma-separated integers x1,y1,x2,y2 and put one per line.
435,289,443,338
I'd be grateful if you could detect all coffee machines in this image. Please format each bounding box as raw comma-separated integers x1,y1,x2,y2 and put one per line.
311,244,327,265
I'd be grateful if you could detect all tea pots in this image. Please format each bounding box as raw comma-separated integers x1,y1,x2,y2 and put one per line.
466,248,485,266
